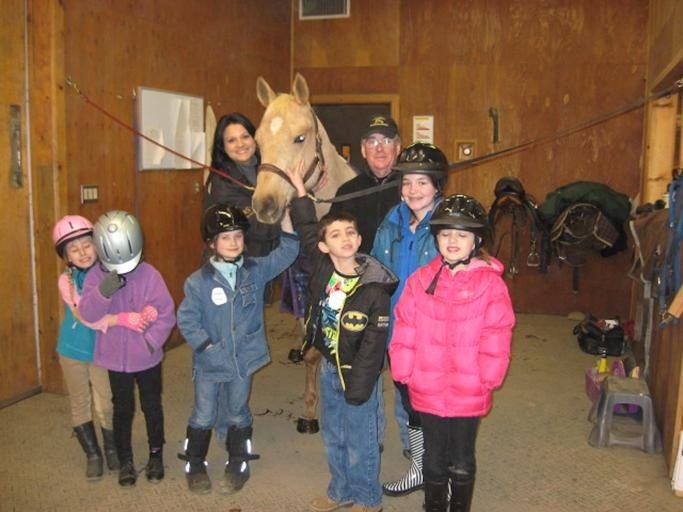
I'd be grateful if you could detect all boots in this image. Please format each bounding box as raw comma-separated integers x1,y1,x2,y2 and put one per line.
217,425,254,495
422,477,450,511
382,420,430,498
447,479,475,511
183,425,214,496
99,425,121,472
72,420,105,481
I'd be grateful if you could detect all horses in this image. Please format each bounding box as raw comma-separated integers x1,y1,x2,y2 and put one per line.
251,73,359,434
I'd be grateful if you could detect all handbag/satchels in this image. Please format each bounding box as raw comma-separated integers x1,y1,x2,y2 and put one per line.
573,311,629,359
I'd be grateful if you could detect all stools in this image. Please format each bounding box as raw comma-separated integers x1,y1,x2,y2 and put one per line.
587,377,656,453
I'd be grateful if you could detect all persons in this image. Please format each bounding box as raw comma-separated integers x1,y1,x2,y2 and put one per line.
364,142,452,505
175,204,302,495
76,210,177,489
282,159,398,512
201,111,282,449
327,117,417,461
386,193,516,512
53,213,160,481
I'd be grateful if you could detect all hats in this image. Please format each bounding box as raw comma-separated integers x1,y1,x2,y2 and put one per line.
360,110,400,139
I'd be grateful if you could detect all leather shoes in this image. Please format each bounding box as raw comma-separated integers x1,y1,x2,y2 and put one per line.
309,496,355,512
351,504,385,512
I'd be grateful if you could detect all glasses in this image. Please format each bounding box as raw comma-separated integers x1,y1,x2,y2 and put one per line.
362,135,396,148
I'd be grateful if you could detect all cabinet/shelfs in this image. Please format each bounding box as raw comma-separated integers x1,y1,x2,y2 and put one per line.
628,0,683,497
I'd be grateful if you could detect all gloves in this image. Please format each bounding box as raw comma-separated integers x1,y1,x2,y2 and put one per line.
114,311,146,333
142,305,160,322
99,268,122,297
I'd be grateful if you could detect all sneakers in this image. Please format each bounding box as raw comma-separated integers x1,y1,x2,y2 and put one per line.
147,451,166,483
117,453,137,487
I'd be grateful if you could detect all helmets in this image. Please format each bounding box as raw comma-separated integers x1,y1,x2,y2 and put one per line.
51,214,94,249
92,209,144,274
199,204,250,240
390,141,450,178
426,193,490,231
